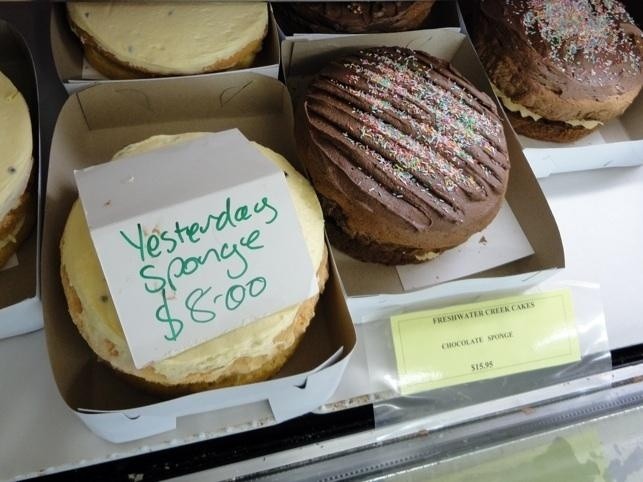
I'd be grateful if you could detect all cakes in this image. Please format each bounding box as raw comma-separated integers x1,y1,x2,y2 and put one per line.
57,132,332,398
57,0,271,79
0,69,35,267
297,44,510,267
284,0,440,33
467,0,643,144
